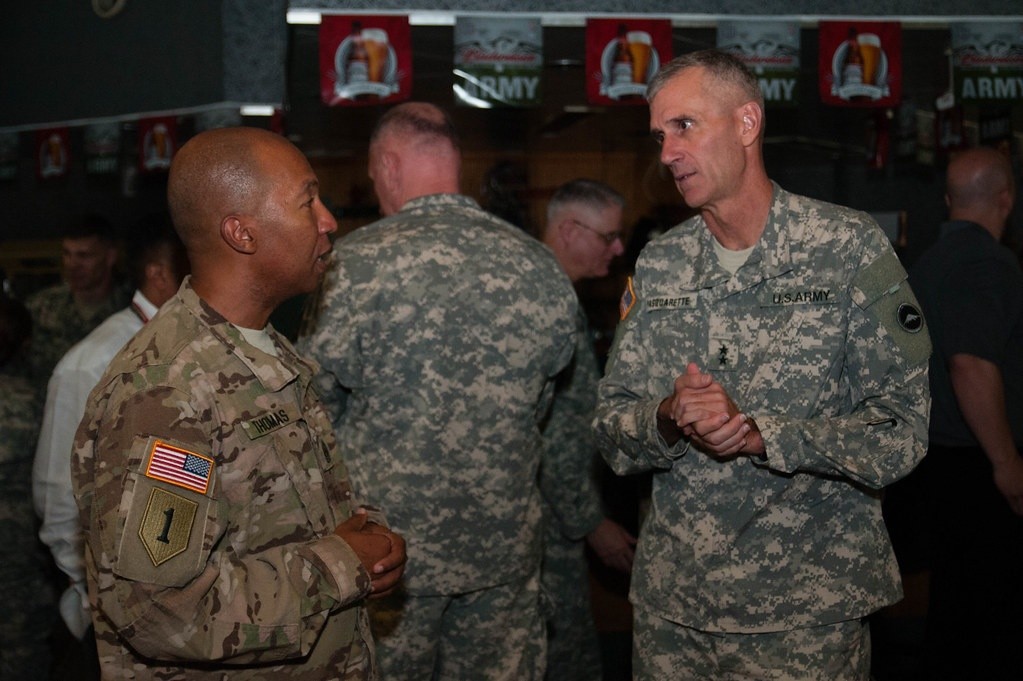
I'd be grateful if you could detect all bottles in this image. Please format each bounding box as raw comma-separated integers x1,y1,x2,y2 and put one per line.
611,24,634,86
839,26,864,87
344,20,367,84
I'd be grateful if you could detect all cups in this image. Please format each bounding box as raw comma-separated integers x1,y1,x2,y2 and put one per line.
626,30,652,85
856,33,881,87
361,27,388,83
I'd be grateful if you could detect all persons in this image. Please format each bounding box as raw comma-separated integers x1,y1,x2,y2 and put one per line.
588,49,935,681
904,146,1023,681
0,102,638,681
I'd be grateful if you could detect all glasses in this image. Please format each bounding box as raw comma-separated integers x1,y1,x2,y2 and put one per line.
573,219,623,245
